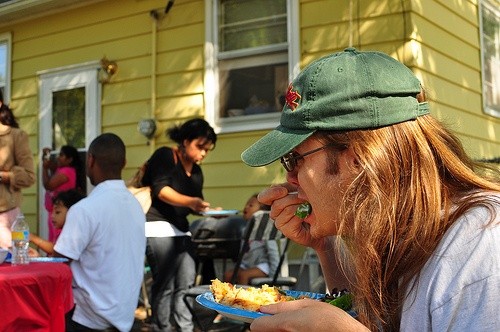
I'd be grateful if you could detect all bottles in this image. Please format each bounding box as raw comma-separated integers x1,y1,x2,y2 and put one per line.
10,212,30,266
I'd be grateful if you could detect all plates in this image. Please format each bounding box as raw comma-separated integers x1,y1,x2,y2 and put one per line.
7,257,71,263
201,209,238,217
195,288,363,325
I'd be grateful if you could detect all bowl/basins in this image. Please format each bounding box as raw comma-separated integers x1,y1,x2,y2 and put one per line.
229,108,244,118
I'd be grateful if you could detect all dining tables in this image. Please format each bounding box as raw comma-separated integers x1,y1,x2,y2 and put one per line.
1,260,74,331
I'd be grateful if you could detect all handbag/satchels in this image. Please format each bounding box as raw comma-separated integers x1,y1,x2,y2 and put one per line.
127,165,152,214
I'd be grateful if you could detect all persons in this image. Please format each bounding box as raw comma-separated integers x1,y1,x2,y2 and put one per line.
29,189,86,258
241,48,500,332
42,144,76,244
50,133,146,331
0,89,35,249
224,193,280,286
128,118,230,331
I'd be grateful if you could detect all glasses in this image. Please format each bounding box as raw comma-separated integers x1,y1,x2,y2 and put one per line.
281,143,338,172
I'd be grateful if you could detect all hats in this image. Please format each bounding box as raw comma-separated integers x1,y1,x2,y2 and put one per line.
241,47,430,167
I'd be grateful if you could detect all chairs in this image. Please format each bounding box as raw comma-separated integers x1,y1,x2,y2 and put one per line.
183,212,293,330
